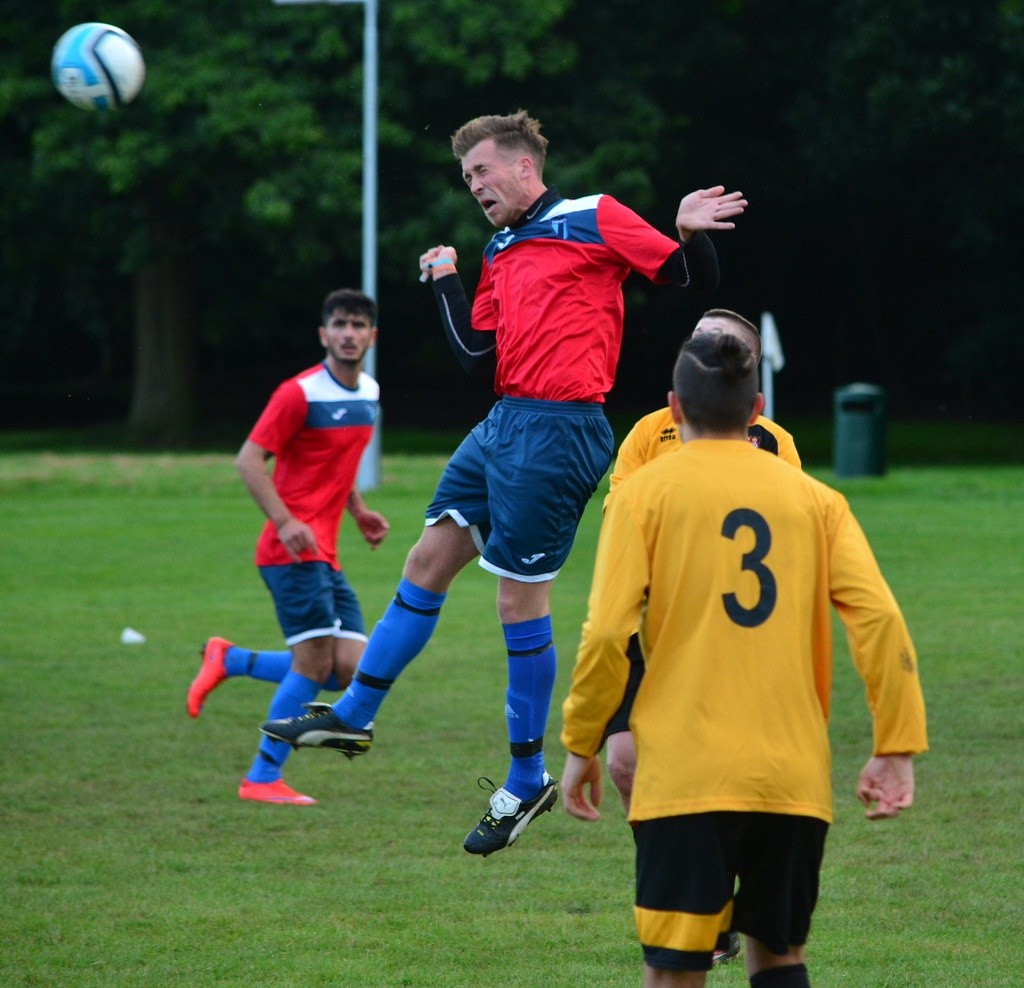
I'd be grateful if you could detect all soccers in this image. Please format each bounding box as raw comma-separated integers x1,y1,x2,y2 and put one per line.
50,23,145,114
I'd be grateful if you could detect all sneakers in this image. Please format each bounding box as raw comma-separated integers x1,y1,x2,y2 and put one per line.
463,769,559,857
239,778,316,807
258,700,376,758
712,929,742,963
187,636,234,719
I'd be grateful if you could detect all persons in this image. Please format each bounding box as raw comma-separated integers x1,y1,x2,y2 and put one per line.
258,110,748,854
560,334,930,988
602,307,803,961
185,288,389,805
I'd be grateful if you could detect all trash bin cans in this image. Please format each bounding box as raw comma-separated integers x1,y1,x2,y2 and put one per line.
833,381,887,478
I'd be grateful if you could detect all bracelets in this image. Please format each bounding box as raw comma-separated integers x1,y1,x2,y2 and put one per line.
426,258,456,273
